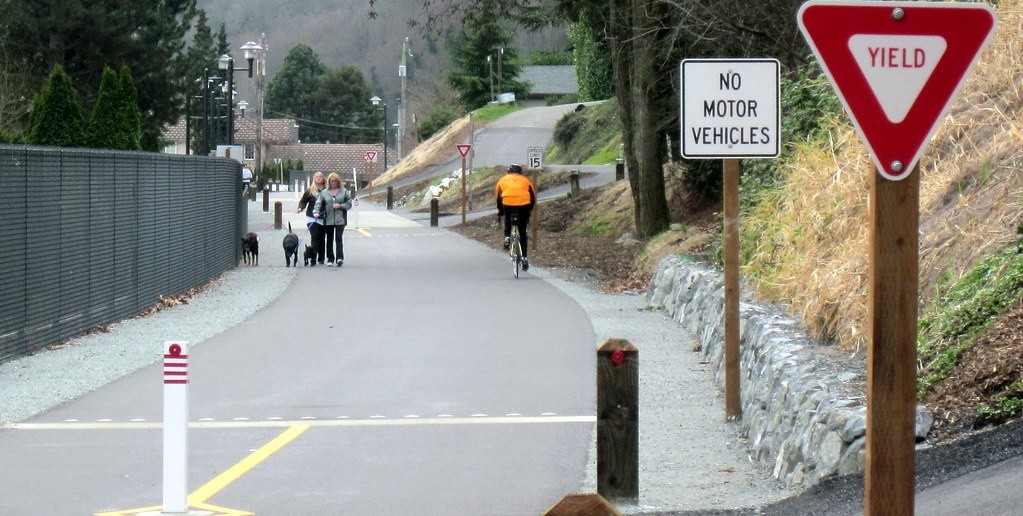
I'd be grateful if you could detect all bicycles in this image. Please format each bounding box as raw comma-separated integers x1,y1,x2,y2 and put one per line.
498,210,527,279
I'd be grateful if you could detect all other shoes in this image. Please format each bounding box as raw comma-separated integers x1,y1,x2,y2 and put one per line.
327,261,333,266
504,237,510,249
337,259,343,266
521,256,529,271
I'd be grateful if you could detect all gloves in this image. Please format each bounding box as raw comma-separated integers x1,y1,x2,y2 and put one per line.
497,210,504,216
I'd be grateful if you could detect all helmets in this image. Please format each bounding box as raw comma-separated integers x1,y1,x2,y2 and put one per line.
508,164,522,175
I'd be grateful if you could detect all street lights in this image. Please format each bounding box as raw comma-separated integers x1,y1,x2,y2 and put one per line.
370,96,388,173
184,53,249,157
226,40,263,147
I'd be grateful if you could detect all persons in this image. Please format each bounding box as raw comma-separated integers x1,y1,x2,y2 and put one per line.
297,171,354,266
242,164,252,190
495,164,537,270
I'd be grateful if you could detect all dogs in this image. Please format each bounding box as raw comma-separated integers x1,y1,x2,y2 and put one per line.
303,244,316,265
282,221,298,267
354,196,360,206
241,232,259,266
